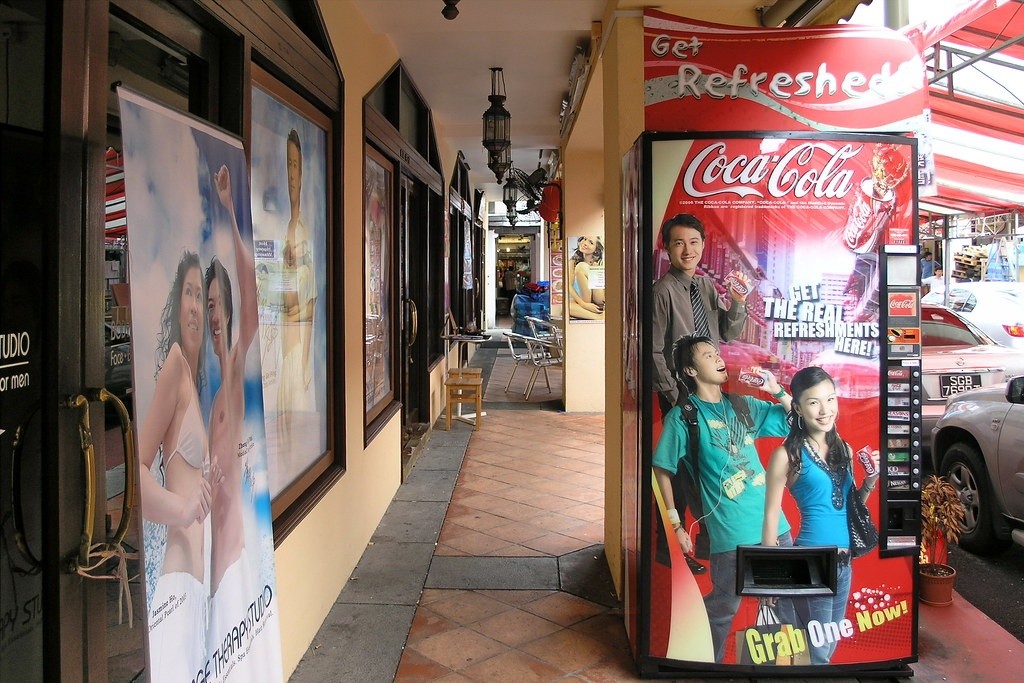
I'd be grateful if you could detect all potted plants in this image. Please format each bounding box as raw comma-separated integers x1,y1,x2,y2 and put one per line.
918,473,966,608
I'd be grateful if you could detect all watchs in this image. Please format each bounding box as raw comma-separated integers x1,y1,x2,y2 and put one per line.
772,383,786,399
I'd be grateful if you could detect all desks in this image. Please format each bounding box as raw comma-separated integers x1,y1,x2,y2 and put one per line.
442,328,492,425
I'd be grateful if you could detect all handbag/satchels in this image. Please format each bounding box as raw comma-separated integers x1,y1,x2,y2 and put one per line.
735,600,811,665
841,435,877,558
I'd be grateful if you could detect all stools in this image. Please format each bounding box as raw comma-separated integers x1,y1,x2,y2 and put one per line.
446,366,483,418
444,377,485,432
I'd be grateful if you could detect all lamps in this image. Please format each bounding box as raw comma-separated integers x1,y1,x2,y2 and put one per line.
508,215,519,230
502,205,519,224
500,179,522,216
483,135,514,187
482,67,512,163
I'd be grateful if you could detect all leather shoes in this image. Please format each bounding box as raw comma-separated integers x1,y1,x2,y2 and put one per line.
683,551,706,575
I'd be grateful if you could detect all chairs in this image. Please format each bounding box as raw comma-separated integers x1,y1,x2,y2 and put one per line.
502,331,551,394
521,337,565,403
524,315,573,392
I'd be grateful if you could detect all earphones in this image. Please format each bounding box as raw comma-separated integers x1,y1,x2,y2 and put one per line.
690,370,695,374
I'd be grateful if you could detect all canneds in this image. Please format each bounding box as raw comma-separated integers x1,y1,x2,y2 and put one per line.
856,445,879,478
842,176,895,255
738,368,768,387
724,270,755,298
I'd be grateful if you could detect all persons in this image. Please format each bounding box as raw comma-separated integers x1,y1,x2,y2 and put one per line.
652,214,756,425
139,253,212,682
761,366,883,664
204,165,257,675
568,235,605,321
271,128,321,484
650,331,794,663
920,252,975,306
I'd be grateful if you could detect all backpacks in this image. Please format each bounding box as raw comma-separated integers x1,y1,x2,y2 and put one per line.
675,391,755,525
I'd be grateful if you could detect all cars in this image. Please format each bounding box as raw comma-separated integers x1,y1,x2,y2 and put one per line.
921,280,1024,344
930,377,1024,557
921,338,1024,469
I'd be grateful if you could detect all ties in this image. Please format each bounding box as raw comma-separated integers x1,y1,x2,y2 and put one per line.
689,278,711,340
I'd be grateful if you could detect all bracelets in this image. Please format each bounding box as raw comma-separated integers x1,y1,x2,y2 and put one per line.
673,525,683,532
862,480,877,493
666,509,682,523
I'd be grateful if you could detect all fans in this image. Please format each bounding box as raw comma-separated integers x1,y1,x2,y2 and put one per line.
505,160,548,207
